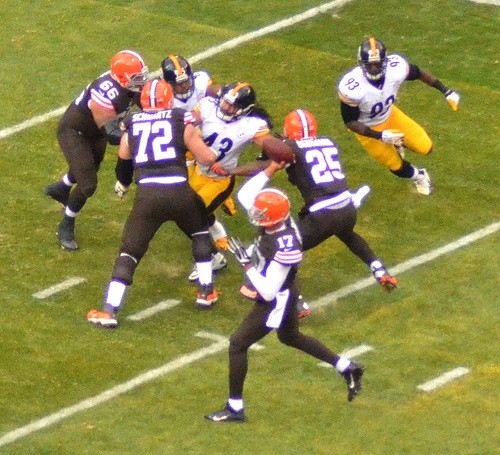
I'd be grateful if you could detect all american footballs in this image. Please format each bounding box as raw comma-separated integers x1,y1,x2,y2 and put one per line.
206,84,221,98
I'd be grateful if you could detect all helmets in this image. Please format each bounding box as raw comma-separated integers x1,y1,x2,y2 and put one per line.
356,38,387,79
159,54,195,99
248,187,290,226
216,82,256,123
140,79,174,112
284,110,318,140
110,50,150,93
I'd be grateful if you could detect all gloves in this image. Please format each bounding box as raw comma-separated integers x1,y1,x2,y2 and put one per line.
381,129,405,148
115,180,130,198
444,88,460,111
227,236,251,266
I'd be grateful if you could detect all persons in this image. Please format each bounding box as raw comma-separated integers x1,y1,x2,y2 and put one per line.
203,153,366,424
158,52,275,282
208,109,397,320
337,37,460,196
86,79,218,327
43,50,150,251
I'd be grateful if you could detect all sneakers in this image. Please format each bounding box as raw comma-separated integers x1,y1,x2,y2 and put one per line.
195,279,220,305
399,145,405,158
188,252,228,280
373,267,398,293
342,361,365,402
415,167,432,196
86,309,118,327
203,402,245,423
296,295,311,319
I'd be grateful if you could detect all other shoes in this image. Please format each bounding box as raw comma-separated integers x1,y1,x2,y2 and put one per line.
45,181,72,208
212,236,230,251
57,218,79,250
220,195,237,215
